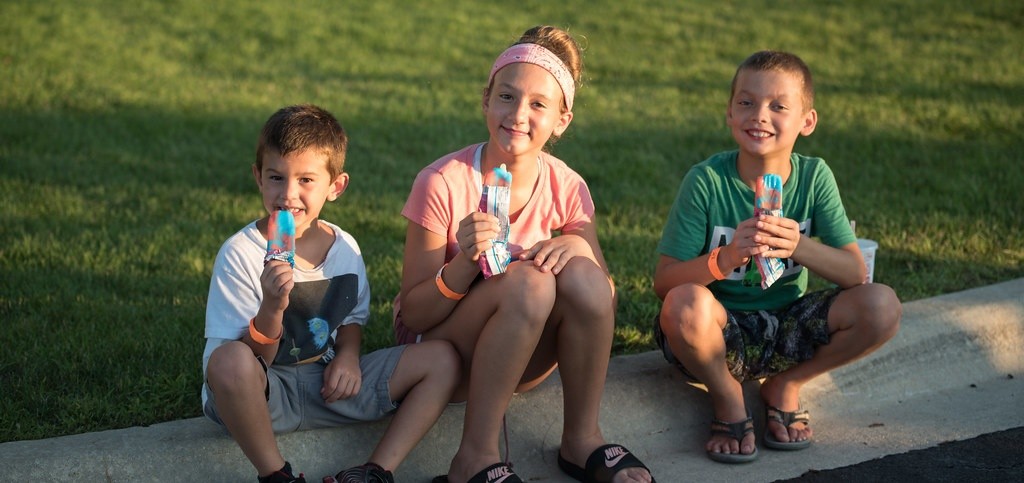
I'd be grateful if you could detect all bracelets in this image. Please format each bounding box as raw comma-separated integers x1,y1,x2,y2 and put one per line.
436,264,469,300
708,246,726,280
249,317,284,344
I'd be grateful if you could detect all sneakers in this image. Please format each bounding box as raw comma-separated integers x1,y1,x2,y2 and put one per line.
322,462,395,483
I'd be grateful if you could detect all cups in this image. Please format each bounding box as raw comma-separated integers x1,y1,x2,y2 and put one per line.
856,239,878,283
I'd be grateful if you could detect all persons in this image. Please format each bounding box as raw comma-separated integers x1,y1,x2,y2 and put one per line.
201,105,464,483
393,25,657,483
652,51,903,461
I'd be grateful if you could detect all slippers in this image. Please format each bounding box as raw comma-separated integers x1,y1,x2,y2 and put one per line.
707,406,759,464
431,463,523,483
557,444,657,483
762,399,812,452
